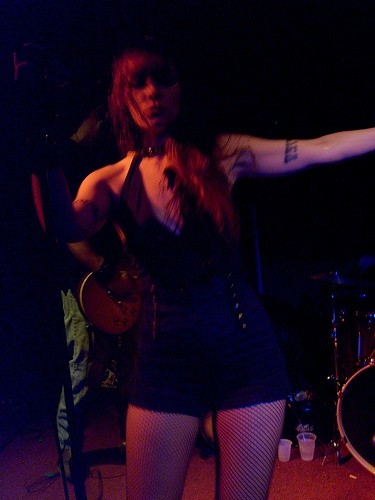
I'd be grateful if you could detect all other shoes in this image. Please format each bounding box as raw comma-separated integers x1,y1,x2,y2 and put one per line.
57,458,72,479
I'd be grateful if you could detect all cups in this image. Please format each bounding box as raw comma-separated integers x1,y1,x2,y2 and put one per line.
296,433,317,461
278,439,293,462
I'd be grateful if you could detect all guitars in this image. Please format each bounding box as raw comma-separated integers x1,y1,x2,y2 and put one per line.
81,251,153,335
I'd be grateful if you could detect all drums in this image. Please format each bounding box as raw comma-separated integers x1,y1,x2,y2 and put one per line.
337,360,375,475
340,308,375,382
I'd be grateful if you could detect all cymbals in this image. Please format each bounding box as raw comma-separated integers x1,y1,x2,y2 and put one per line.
311,272,375,288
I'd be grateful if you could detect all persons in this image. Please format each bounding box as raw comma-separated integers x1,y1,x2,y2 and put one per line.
32,85,130,487
62,37,374,500
